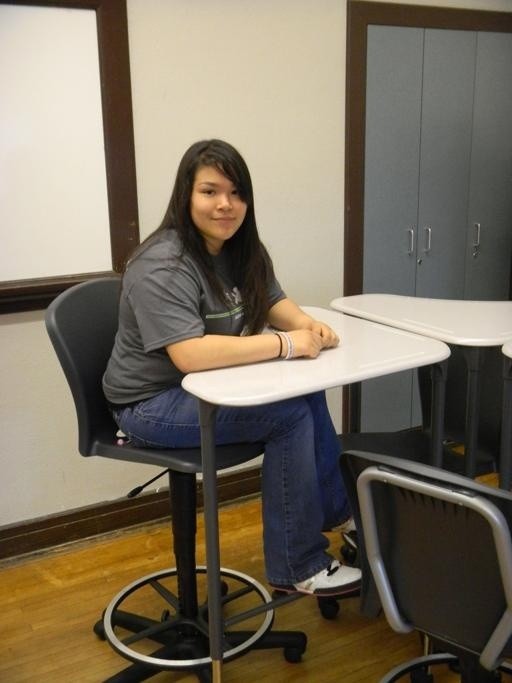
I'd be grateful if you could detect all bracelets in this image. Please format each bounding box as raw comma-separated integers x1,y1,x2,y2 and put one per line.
273,326,294,365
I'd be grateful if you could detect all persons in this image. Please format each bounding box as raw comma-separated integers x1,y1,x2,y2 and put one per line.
102,138,369,596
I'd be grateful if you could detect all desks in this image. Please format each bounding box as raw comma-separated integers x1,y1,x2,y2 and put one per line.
331,292,506,500
181,303,451,681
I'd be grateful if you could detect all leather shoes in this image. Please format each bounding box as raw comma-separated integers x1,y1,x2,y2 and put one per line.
269,559,362,596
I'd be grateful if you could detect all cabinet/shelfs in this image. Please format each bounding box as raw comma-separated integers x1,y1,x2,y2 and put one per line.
358,20,510,436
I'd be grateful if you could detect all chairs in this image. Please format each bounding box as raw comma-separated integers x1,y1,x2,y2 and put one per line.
43,274,307,681
337,448,511,683
